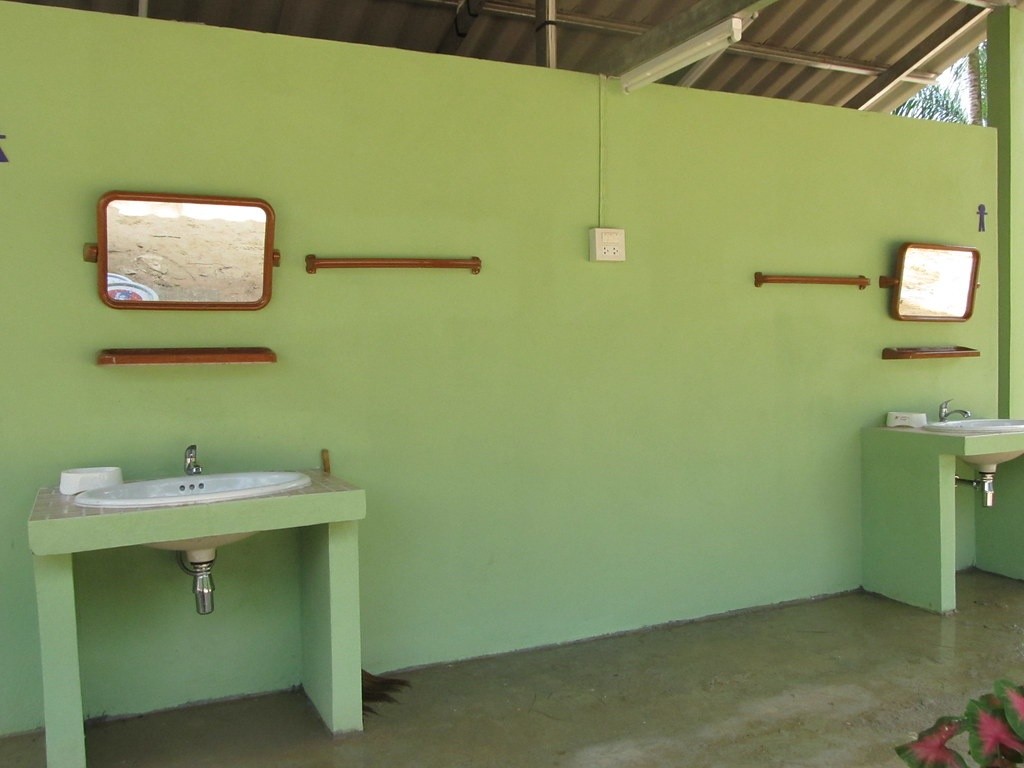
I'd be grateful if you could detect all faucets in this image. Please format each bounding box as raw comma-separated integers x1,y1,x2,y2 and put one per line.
938,398,971,422
182,444,203,476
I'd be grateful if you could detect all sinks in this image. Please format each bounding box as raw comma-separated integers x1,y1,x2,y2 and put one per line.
921,418,1024,466
73,471,312,551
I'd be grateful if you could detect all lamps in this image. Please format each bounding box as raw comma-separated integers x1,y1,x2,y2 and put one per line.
619,18,743,95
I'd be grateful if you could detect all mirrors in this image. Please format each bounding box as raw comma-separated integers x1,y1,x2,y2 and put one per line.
897,249,976,318
106,200,267,305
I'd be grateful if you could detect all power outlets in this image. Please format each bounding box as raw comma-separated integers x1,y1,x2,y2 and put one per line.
588,227,626,260
